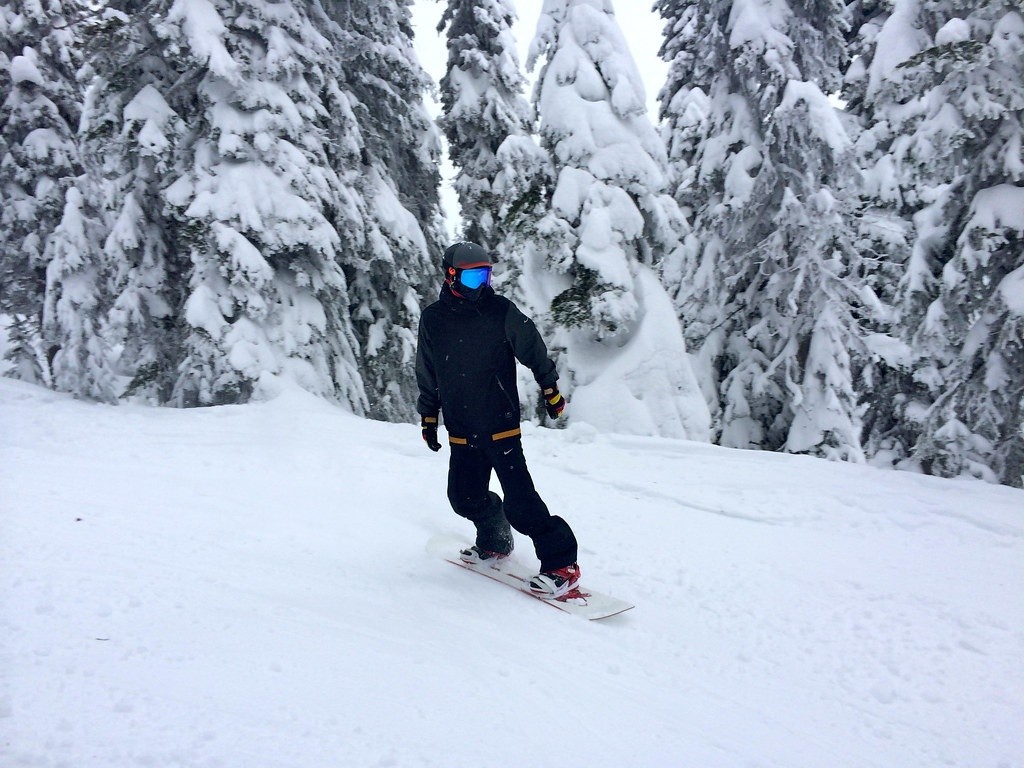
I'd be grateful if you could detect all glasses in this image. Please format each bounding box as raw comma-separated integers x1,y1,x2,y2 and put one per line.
455,266,488,289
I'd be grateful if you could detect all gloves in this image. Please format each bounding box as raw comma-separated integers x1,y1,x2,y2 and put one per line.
542,382,566,421
422,410,442,452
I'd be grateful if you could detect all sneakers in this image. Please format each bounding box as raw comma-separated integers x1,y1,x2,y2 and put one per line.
460,538,514,565
530,562,580,598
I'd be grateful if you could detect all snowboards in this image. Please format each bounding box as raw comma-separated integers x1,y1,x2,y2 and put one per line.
427,534,633,621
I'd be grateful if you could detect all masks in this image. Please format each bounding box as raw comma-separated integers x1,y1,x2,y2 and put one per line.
451,283,488,301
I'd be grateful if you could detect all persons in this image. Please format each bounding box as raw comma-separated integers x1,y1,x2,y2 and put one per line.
415,242,579,600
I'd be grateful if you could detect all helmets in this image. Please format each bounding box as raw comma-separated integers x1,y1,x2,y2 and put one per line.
439,242,493,270
